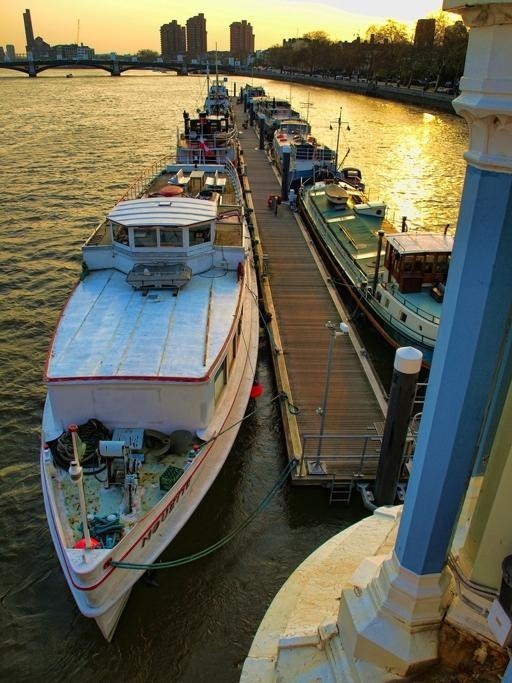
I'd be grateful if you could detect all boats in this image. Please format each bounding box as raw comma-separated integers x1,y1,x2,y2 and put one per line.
173,41,365,189
296,162,456,384
36,145,263,643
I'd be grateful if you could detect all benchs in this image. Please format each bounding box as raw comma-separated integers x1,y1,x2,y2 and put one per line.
168,168,227,194
